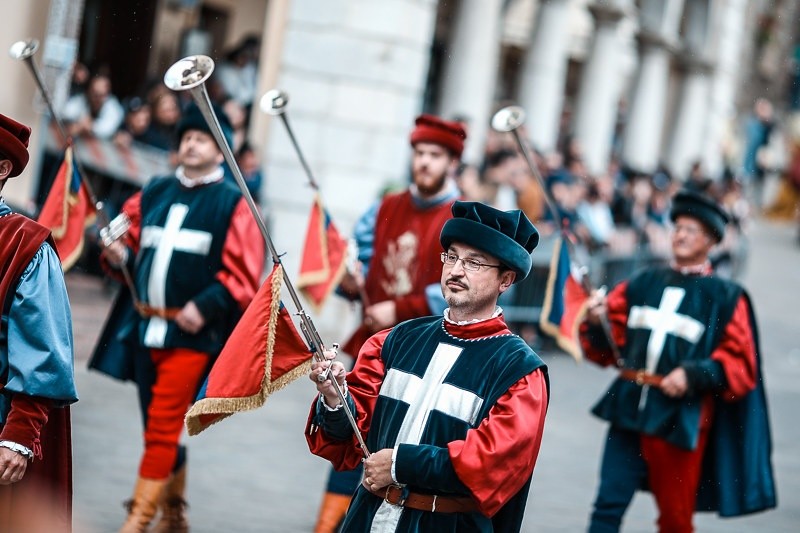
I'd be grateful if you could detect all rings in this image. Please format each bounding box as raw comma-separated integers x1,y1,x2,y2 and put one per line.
365,478,371,485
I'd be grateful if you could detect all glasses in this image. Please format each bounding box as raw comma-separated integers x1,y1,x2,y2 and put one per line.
440,252,507,271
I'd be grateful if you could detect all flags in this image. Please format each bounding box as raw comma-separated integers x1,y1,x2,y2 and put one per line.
36,147,97,272
184,262,313,437
294,200,349,315
540,234,601,366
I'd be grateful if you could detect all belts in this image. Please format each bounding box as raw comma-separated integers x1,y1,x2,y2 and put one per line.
134,302,180,318
361,474,475,512
619,368,662,386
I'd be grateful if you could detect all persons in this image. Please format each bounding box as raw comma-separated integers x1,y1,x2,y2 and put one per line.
305,199,550,533
100,102,268,533
0,113,78,533
335,112,467,372
454,144,613,347
611,152,748,259
36,59,263,278
587,188,779,533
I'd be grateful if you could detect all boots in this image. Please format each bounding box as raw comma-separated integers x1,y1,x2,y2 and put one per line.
313,491,352,533
152,447,188,533
120,476,174,533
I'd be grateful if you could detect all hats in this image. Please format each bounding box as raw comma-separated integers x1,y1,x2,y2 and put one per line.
439,200,539,284
669,191,731,244
411,114,466,158
0,113,31,177
171,100,236,153
